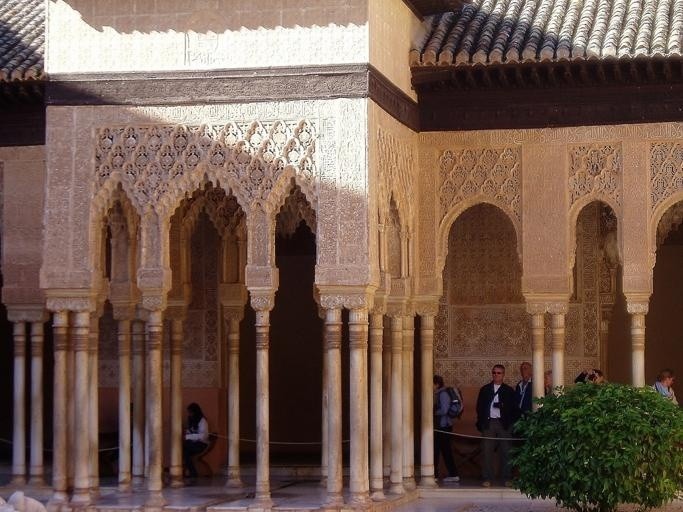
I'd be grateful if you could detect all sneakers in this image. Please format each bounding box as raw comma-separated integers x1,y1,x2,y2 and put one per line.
482,480,491,487
505,480,513,487
442,476,460,482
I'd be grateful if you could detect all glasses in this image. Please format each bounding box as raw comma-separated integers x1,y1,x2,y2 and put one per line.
492,371,502,375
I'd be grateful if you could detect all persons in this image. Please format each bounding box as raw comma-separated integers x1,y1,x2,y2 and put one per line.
180,401,210,479
473,363,519,488
543,368,552,396
573,368,603,384
432,373,461,482
512,359,532,414
651,368,679,406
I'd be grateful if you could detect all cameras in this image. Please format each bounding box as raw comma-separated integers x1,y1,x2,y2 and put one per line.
588,374,596,380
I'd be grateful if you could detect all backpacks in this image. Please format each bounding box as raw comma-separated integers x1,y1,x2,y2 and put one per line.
437,385,464,418
